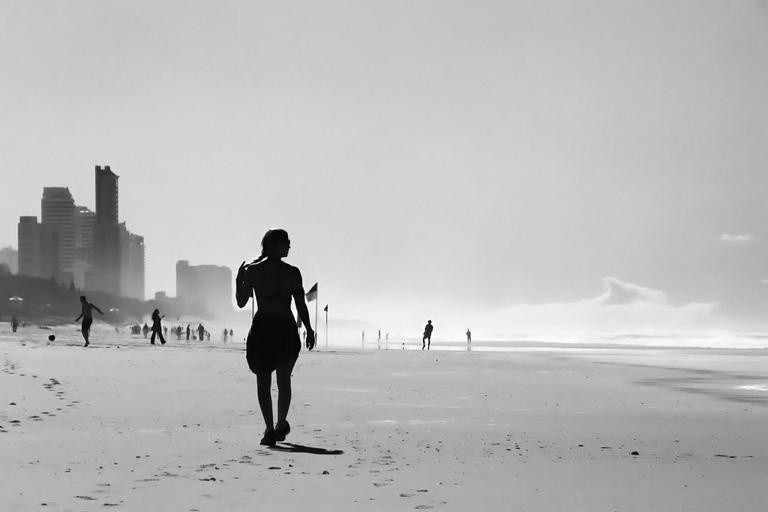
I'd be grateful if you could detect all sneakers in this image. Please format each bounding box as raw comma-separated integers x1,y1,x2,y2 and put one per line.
260,420,290,447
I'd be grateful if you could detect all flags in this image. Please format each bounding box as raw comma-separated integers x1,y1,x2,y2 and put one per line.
305,282,317,302
324,305,328,312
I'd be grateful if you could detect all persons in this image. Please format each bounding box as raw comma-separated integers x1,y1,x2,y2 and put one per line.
422,320,433,351
466,329,471,344
151,309,166,345
11,316,18,332
75,296,104,347
236,229,315,444
223,329,228,343
129,322,210,342
229,329,233,336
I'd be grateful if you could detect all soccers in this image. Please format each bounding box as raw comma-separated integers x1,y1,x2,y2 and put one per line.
49,334,54,341
